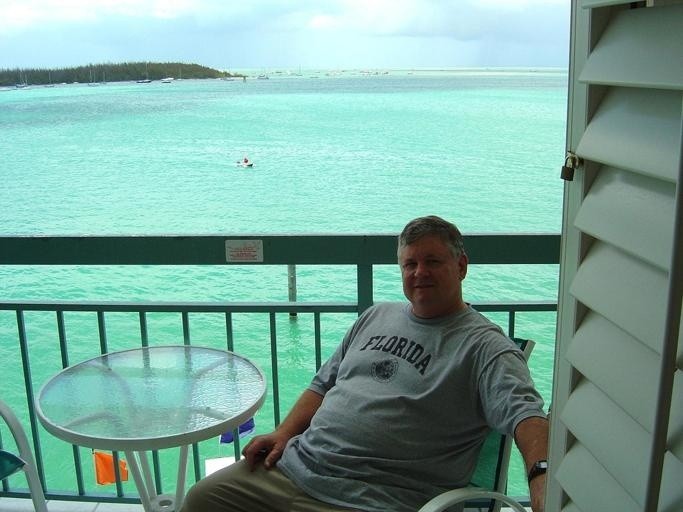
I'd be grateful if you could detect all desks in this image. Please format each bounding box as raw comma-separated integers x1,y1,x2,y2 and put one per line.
34,345,266,512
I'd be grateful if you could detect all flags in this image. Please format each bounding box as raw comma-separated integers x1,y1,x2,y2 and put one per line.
94,451,128,486
219,418,256,445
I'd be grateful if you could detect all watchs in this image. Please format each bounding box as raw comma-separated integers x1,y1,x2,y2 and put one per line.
528,460,547,488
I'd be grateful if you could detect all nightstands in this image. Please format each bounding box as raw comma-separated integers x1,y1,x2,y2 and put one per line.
414,337,535,512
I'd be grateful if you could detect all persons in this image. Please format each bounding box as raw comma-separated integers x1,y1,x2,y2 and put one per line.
181,216,549,512
243,158,249,168
